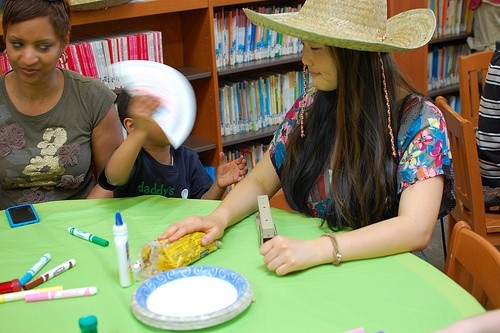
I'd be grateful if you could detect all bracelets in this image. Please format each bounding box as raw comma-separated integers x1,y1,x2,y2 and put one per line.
320,233,341,265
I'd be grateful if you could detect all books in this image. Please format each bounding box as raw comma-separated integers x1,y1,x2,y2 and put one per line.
0,28,163,78
220,70,312,137
428,0,469,38
428,43,471,91
224,144,271,193
447,96,461,114
213,4,304,68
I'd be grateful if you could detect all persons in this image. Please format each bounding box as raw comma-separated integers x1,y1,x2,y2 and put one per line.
158,0,457,276
100,89,248,199
0,0,125,212
467,0,500,50
475,42,500,189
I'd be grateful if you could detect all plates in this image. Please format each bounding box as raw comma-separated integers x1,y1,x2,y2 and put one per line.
129,264,254,331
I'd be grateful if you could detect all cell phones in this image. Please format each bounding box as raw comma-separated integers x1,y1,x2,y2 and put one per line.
7,203,40,228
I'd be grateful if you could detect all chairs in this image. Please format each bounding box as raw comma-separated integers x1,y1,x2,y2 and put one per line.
442,219,500,310
456,48,494,131
435,94,500,250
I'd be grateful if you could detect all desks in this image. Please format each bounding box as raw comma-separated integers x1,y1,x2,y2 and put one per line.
0,197,487,333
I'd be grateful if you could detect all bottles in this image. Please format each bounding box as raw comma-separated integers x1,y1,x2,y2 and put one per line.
0,278,21,294
113,210,135,287
78,315,98,333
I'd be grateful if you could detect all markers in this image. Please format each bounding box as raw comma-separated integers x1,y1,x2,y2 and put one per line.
23,258,77,291
20,254,52,286
0,286,64,303
68,227,109,247
24,286,98,301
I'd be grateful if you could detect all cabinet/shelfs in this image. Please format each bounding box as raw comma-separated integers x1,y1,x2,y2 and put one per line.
0,0,474,211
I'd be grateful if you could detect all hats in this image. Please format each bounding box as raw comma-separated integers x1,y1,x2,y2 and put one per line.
242,0,437,52
